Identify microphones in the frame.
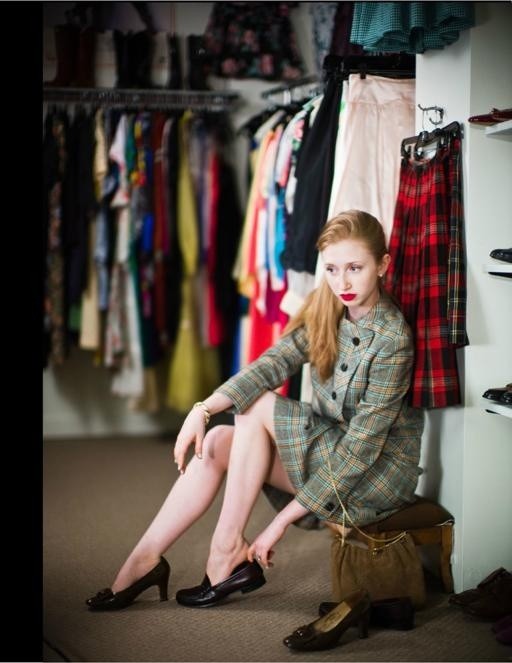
[326,498,453,595]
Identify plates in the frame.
[482,119,512,420]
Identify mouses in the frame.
[400,107,460,157]
[267,52,416,110]
[47,92,231,117]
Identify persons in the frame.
[86,209,425,612]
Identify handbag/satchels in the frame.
[195,401,210,426]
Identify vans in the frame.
[84,555,171,612]
[282,595,372,652]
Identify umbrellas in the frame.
[490,248,511,263]
[466,107,511,126]
[482,383,512,408]
[447,567,512,652]
[175,559,268,610]
[319,595,416,631]
